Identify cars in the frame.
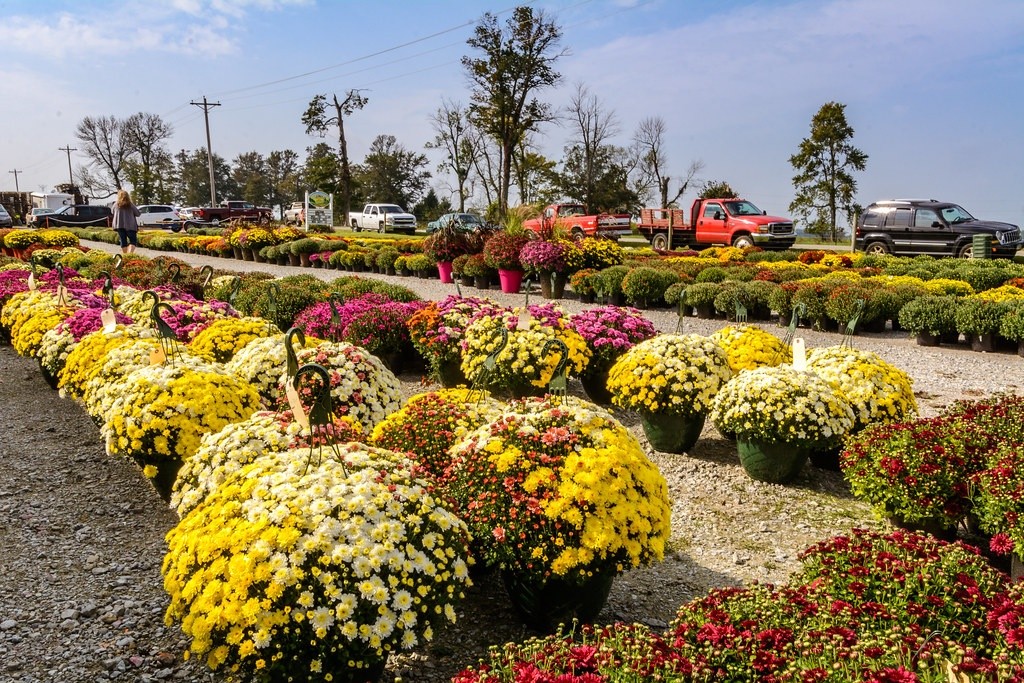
[25,208,54,228]
[425,213,505,236]
[0,203,14,229]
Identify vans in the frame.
[283,202,315,226]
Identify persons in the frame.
[111,191,141,255]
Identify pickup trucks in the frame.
[348,203,418,235]
[521,203,633,243]
[199,200,274,225]
[636,198,797,251]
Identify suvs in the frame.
[850,198,1024,263]
[29,205,114,229]
[135,204,199,232]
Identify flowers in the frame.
[581,361,614,404]
[0,218,1024,683]
[438,359,475,390]
[309,254,319,261]
[508,382,560,401]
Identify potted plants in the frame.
[571,265,1024,351]
[451,253,498,289]
[329,249,429,277]
[437,262,454,283]
[173,235,228,257]
[259,236,320,268]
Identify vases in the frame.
[242,248,253,261]
[503,564,618,636]
[498,269,523,293]
[252,244,266,262]
[133,452,185,505]
[539,267,568,298]
[736,433,804,483]
[641,408,706,454]
[234,247,243,259]
